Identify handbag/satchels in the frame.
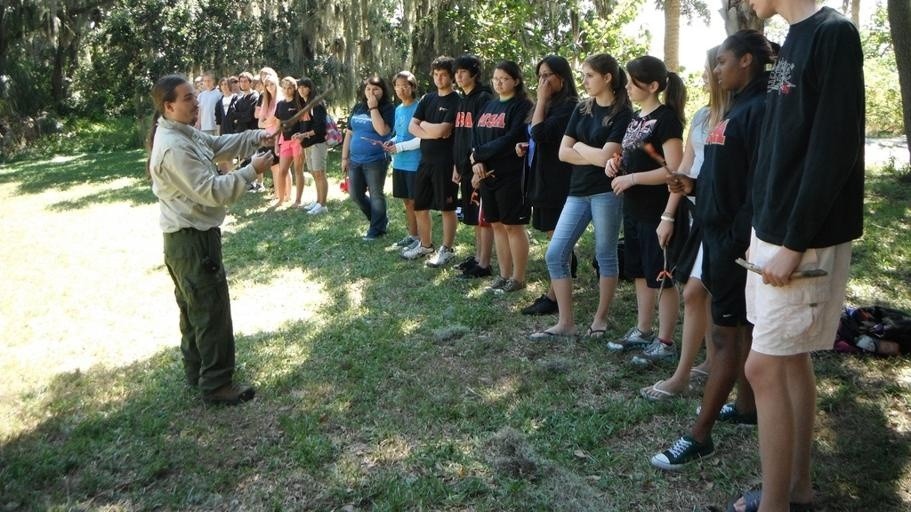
[324,113,342,147]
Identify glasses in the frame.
[491,78,512,82]
[393,85,411,90]
[538,73,554,78]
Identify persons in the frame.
[340,75,389,240]
[637,39,727,402]
[519,54,580,316]
[379,71,422,258]
[724,0,865,512]
[526,54,628,341]
[452,54,491,281]
[146,75,280,407]
[405,54,459,267]
[469,62,532,294]
[149,66,332,219]
[610,56,688,370]
[650,24,775,474]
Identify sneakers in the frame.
[246,182,327,214]
[202,385,253,405]
[362,235,558,315]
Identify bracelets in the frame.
[365,105,379,113]
[657,212,676,223]
